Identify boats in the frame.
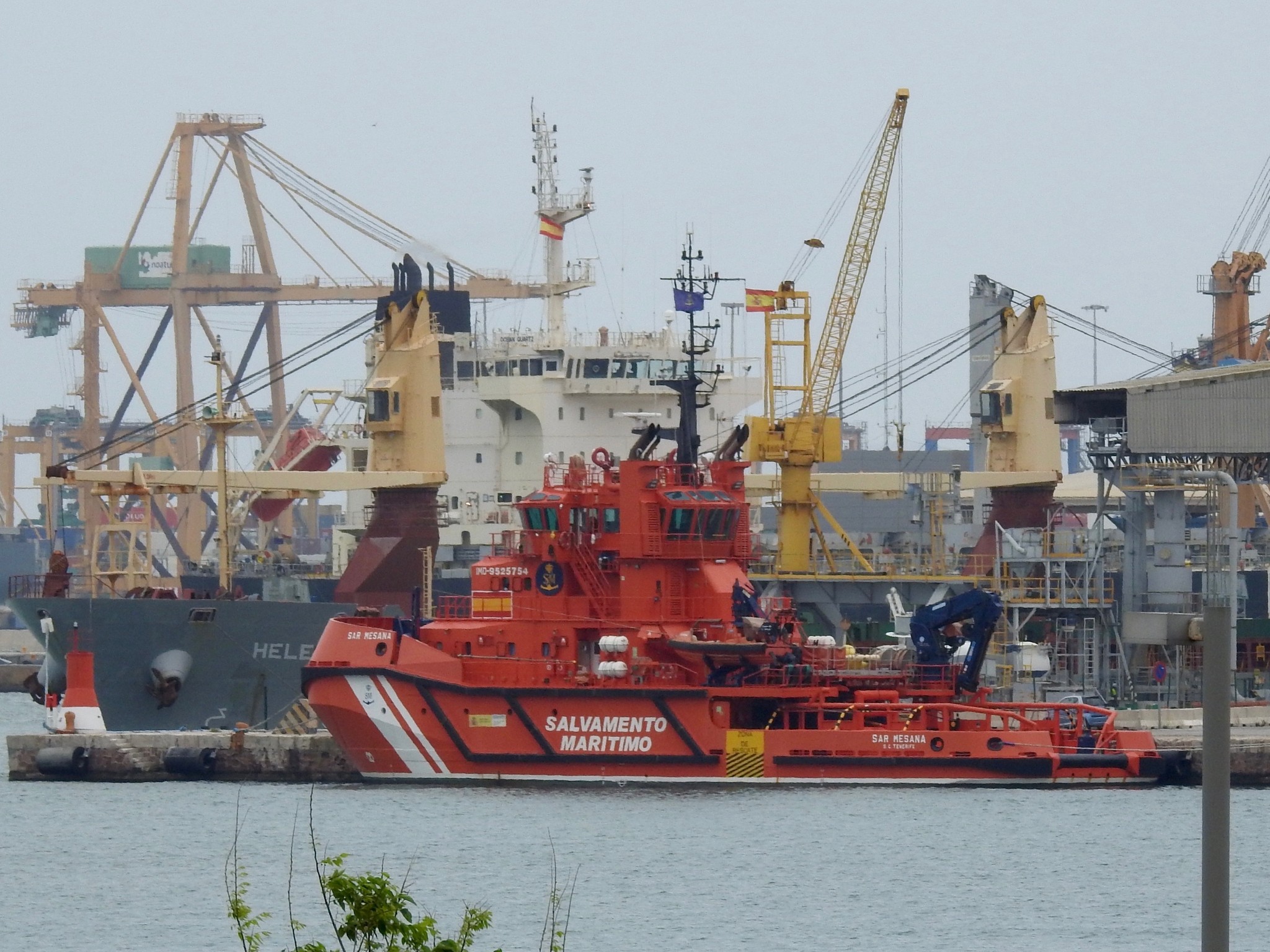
[300,232,1202,791]
[667,596,847,671]
[249,427,340,522]
[0,97,972,732]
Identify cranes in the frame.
[9,109,598,598]
[740,87,912,644]
[909,585,1003,722]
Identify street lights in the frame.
[1080,304,1110,467]
[720,302,745,374]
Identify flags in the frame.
[672,287,704,312]
[745,288,776,312]
[540,216,564,240]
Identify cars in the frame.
[1042,695,1108,730]
